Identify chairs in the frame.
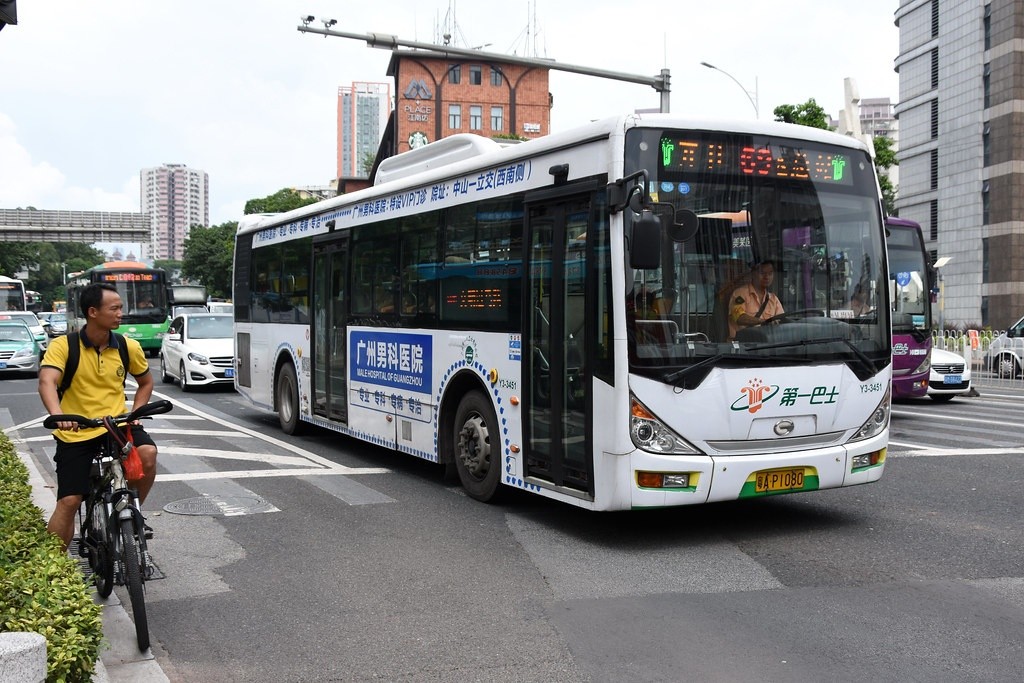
[265,259,416,313]
[715,260,747,343]
[652,288,678,314]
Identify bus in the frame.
[731,210,941,402]
[0,274,29,312]
[63,259,176,357]
[378,255,587,330]
[25,290,44,312]
[232,112,896,515]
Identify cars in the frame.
[158,312,235,392]
[0,300,68,379]
[925,348,971,404]
[157,283,233,325]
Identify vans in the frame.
[984,315,1024,379]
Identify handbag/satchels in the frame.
[122,424,146,480]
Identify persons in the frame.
[335,272,426,328]
[139,294,154,308]
[726,257,785,343]
[841,284,875,318]
[39,285,158,558]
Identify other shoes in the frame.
[134,523,153,539]
